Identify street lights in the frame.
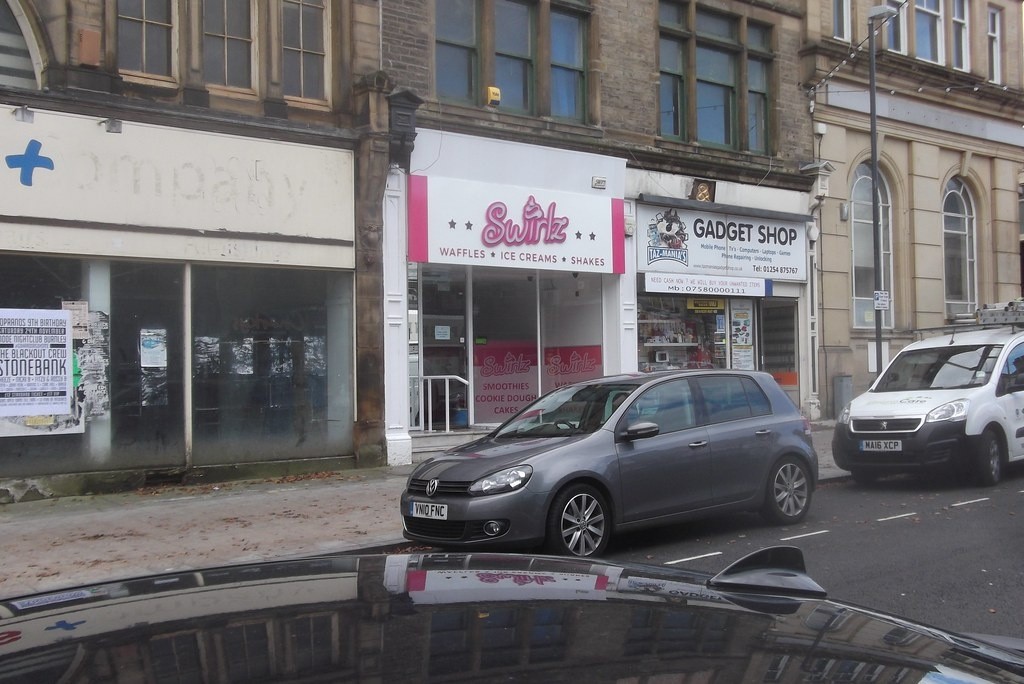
[867,4,899,378]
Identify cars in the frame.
[401,367,818,559]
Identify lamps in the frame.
[97,115,123,134]
[12,104,34,124]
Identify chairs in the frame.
[612,394,636,426]
[653,386,687,431]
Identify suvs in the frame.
[831,301,1024,485]
